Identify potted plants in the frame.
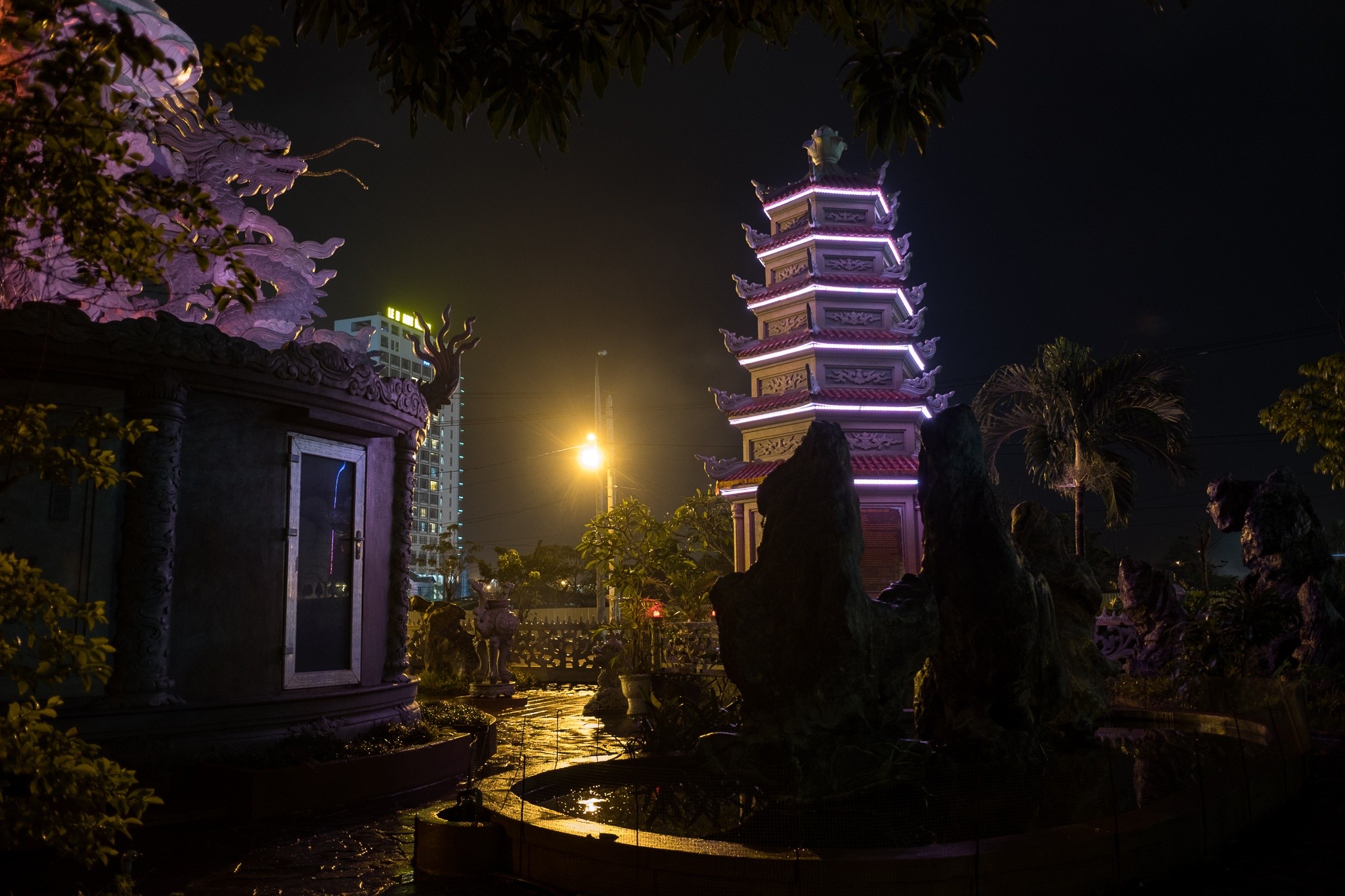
[589,575,672,715]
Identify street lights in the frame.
[580,429,607,618]
